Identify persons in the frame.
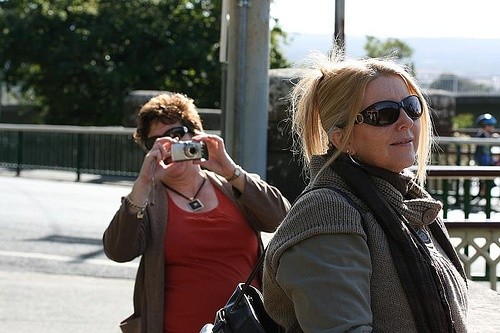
[263,61,468,333]
[102,94,292,333]
[470,115,500,213]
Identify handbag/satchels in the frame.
[201,278,272,333]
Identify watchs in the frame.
[226,166,241,181]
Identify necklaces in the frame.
[161,176,206,211]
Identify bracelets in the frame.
[126,196,148,211]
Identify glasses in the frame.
[144,125,193,150]
[336,94,424,127]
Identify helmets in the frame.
[477,113,497,124]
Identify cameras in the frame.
[171,140,202,162]
